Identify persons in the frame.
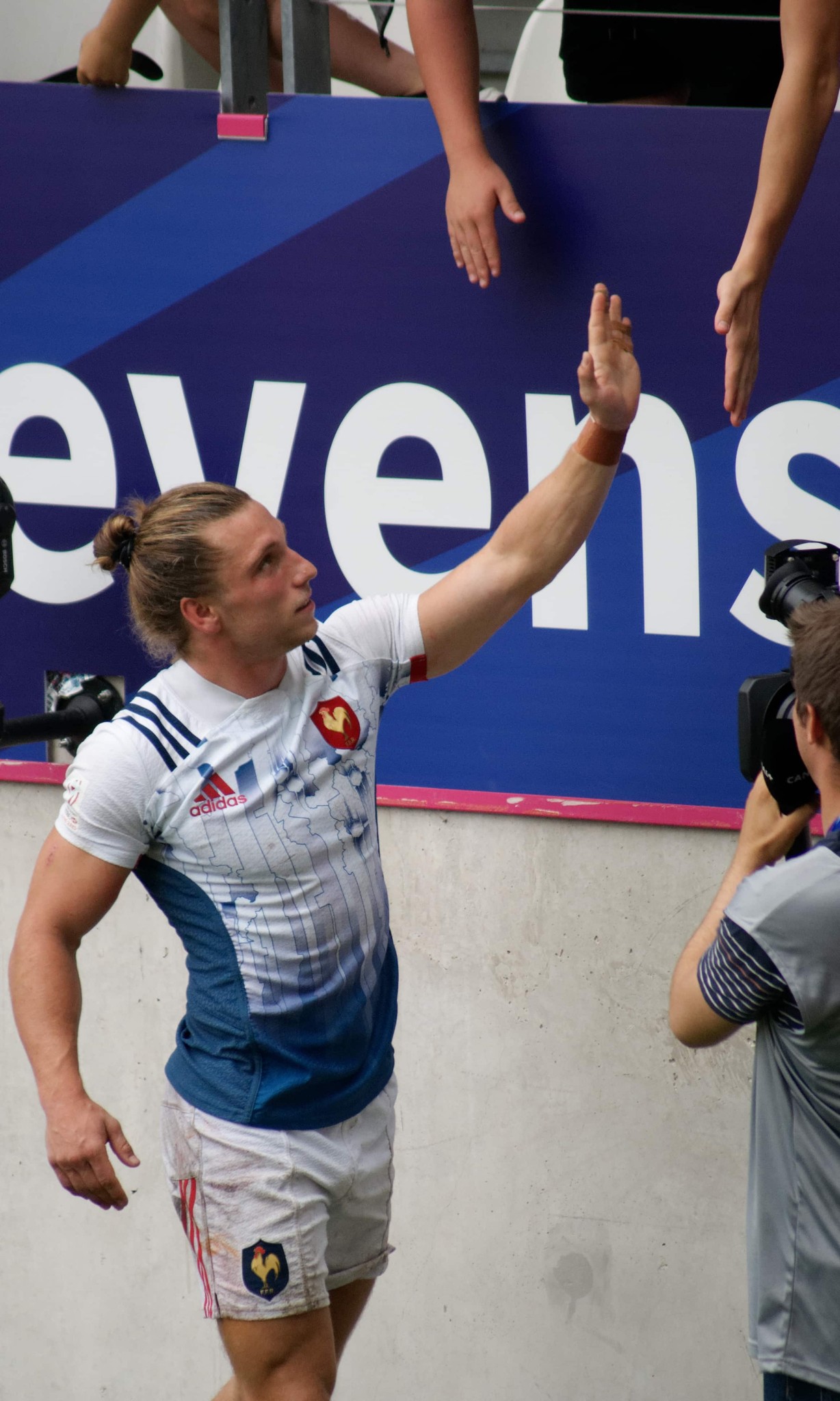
[405,0,840,428]
[9,282,641,1401]
[668,597,840,1401]
[77,0,509,103]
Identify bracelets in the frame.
[574,417,629,466]
